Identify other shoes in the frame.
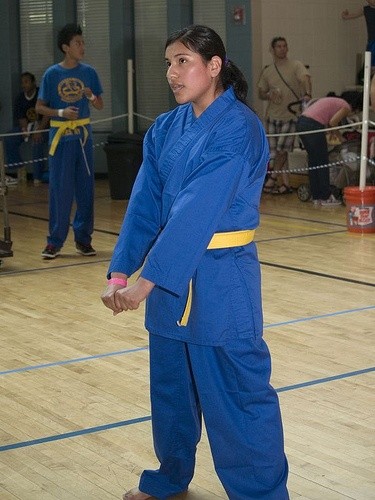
[321,194,342,205]
[34,180,41,184]
[312,199,320,205]
[6,176,19,185]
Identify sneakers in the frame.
[76,241,96,256]
[41,245,60,257]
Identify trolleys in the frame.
[282,97,375,201]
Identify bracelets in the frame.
[304,92,311,97]
[86,93,95,101]
[58,109,63,117]
[265,92,270,98]
[106,277,127,287]
[90,97,97,104]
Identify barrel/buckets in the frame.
[344,188,375,234]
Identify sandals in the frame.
[263,176,277,193]
[272,184,294,195]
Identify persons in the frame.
[296,91,363,205]
[35,24,104,257]
[16,72,50,185]
[100,26,290,500]
[256,37,312,195]
